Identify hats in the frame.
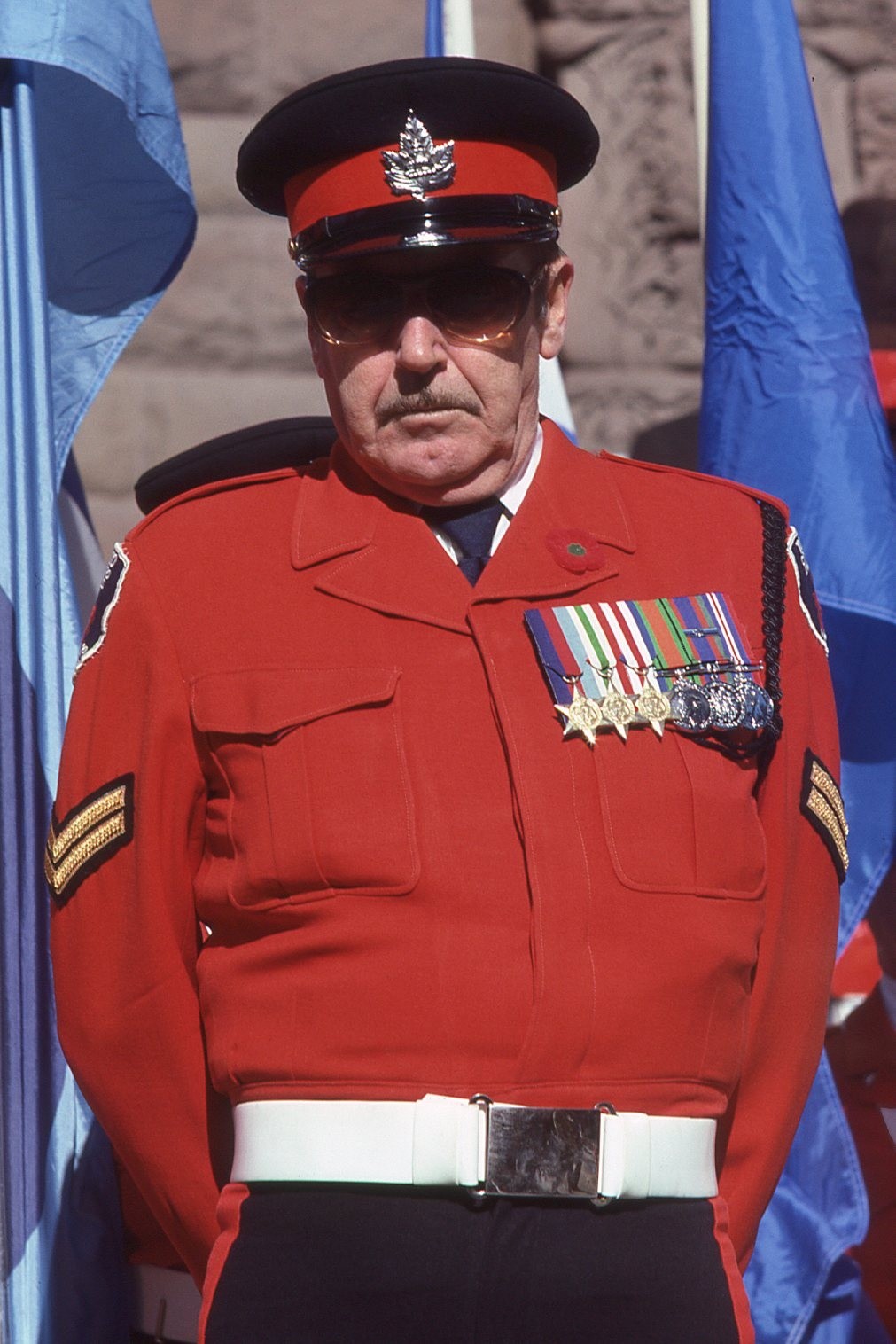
[235,56,600,279]
[134,414,339,514]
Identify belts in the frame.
[227,1094,721,1200]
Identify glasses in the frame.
[304,244,562,346]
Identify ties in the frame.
[420,496,507,585]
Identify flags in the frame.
[699,0,895,1344]
[0,2,202,1343]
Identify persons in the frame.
[0,0,896,1344]
